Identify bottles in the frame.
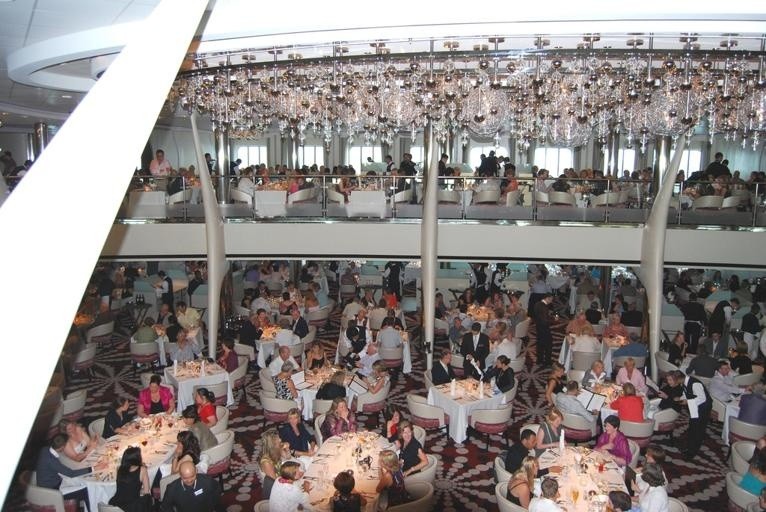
[478,381,485,400]
[224,314,243,329]
[134,293,144,304]
[450,378,456,396]
[558,428,565,451]
[172,359,178,376]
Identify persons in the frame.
[30,258,765,512]
[1,149,33,192]
[531,165,654,203]
[439,150,518,203]
[205,152,417,202]
[126,150,200,205]
[677,152,766,210]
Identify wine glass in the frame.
[570,487,580,510]
